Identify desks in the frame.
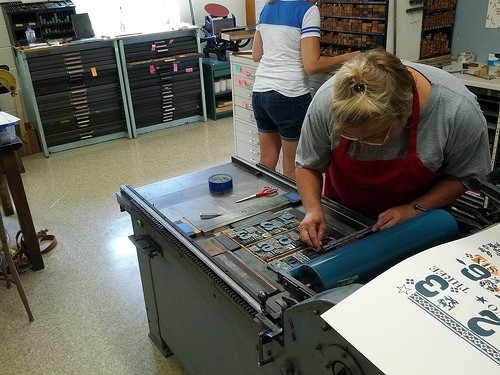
[0,137,45,271]
[432,57,500,209]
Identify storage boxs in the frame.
[15,121,41,156]
[0,111,21,146]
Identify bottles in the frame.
[25,26,36,49]
[456,52,466,65]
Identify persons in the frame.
[294,49,492,253]
[252,0,360,180]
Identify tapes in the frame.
[209,174,232,191]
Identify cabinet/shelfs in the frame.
[201,55,233,120]
[15,38,134,159]
[1,0,77,46]
[119,27,207,135]
[229,49,343,182]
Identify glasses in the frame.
[339,116,397,147]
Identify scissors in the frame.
[236,186,278,203]
